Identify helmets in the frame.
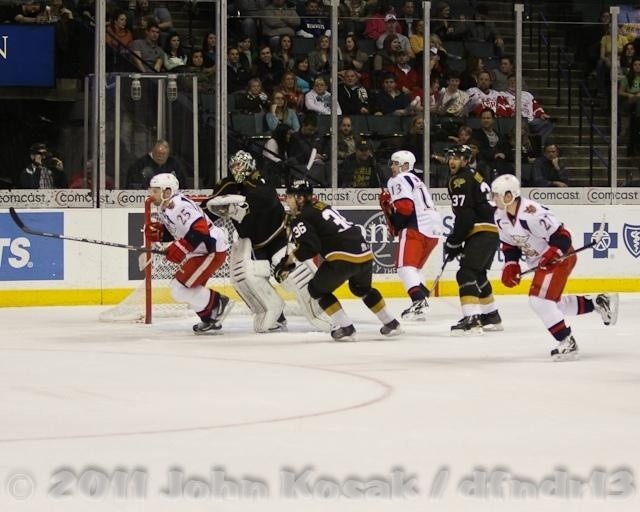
[491,175,521,204]
[150,173,179,201]
[391,151,416,170]
[229,150,257,184]
[285,179,312,194]
[447,144,474,164]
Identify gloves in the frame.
[144,222,165,242]
[538,248,565,272]
[165,238,193,264]
[501,261,520,287]
[443,240,462,261]
[274,255,295,283]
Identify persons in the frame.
[379,150,443,317]
[444,144,501,331]
[150,173,230,332]
[274,180,400,340]
[492,174,611,356]
[199,150,338,333]
[0,0,575,190]
[585,11,640,157]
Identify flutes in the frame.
[10,207,168,254]
[284,148,316,259]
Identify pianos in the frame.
[522,214,607,276]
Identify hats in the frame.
[356,139,372,150]
[29,142,46,150]
[384,12,396,23]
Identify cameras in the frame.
[39,152,57,170]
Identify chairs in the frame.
[73,0,638,188]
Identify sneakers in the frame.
[331,324,355,339]
[451,310,501,330]
[551,335,578,355]
[193,320,223,331]
[401,297,428,317]
[209,295,229,323]
[381,320,399,335]
[595,293,611,325]
[268,316,286,330]
